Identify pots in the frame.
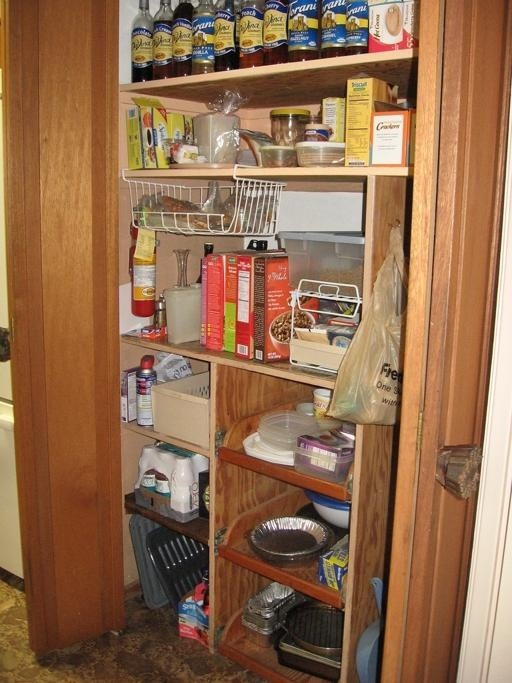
[277,599,345,655]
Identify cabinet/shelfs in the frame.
[118,46,419,683]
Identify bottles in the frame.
[154,301,167,328]
[130,0,369,83]
[196,243,215,285]
[133,441,210,524]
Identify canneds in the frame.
[269,109,310,146]
[304,123,331,141]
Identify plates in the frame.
[385,4,402,36]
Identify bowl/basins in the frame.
[258,142,345,167]
[143,127,154,147]
[303,488,351,530]
[269,311,316,354]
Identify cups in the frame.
[313,388,343,432]
